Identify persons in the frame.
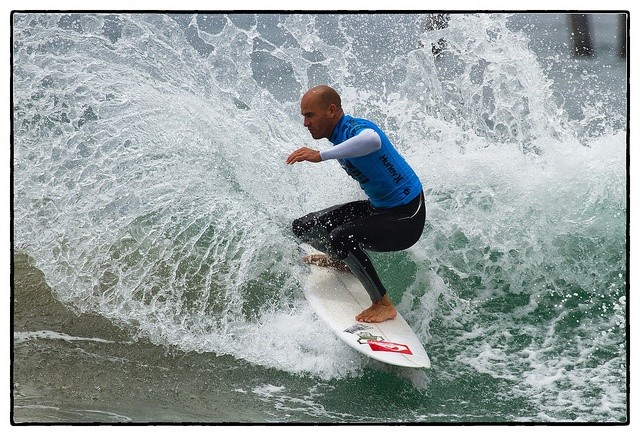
[285,85,425,323]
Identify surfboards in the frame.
[297,242,432,367]
